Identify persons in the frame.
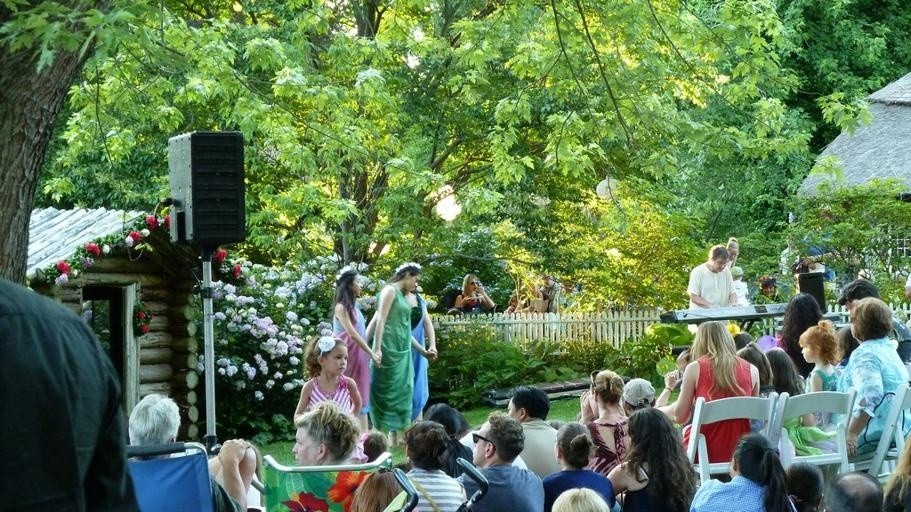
[0,279,127,512]
[128,394,257,512]
[292,235,911,512]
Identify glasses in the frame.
[473,434,496,451]
[591,370,598,387]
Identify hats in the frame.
[623,378,655,407]
[838,279,878,305]
[731,267,744,277]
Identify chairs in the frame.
[855,384,910,487]
[124,443,214,512]
[770,387,857,474]
[686,392,777,485]
[264,451,392,512]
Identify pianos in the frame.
[661,303,789,323]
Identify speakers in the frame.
[798,272,828,314]
[168,131,246,246]
